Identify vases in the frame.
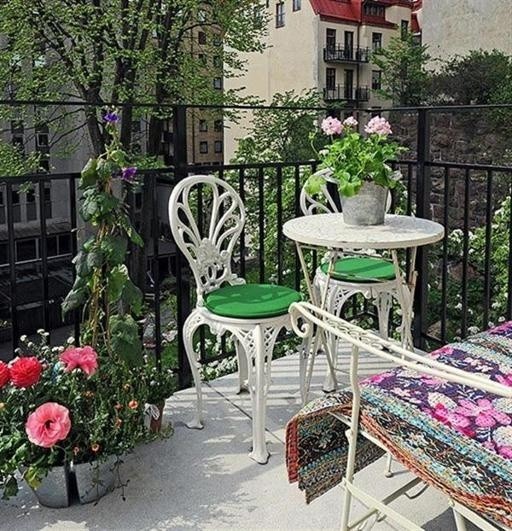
[21,455,121,509]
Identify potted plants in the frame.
[144,367,175,435]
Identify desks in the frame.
[282,214,443,408]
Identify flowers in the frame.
[0,328,142,505]
[316,114,403,180]
